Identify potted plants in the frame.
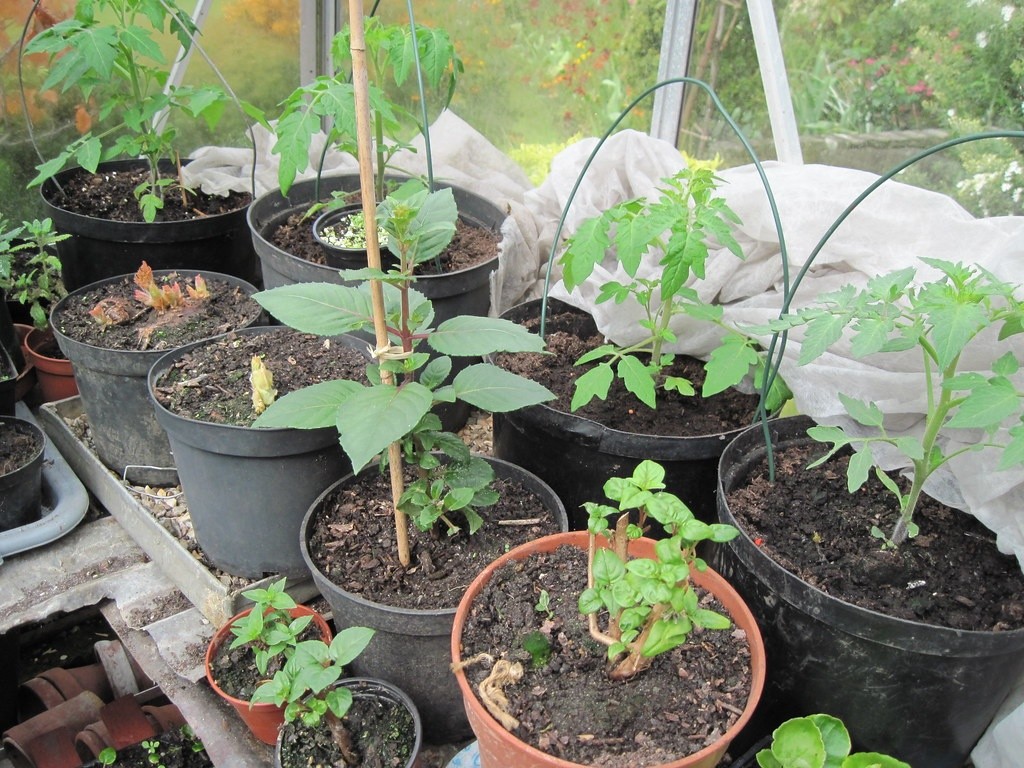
[0,0,1023,768]
